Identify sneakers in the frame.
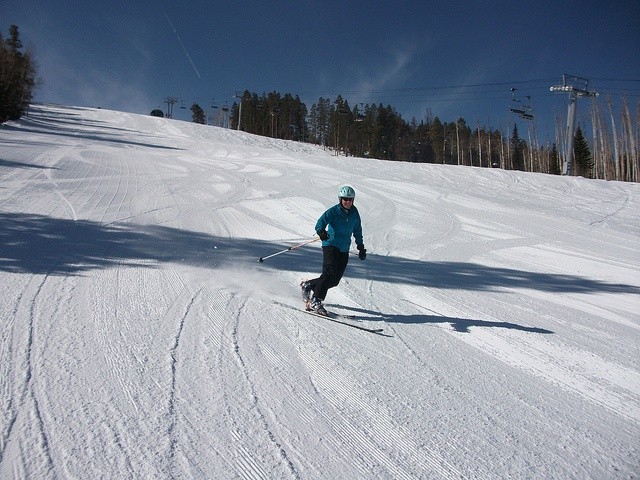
[300,280,311,303]
[312,297,327,315]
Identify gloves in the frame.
[318,230,327,241]
[357,244,366,260]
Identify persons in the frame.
[300,186,367,316]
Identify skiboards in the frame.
[269,293,384,333]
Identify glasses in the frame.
[342,197,354,201]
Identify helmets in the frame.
[338,185,355,202]
